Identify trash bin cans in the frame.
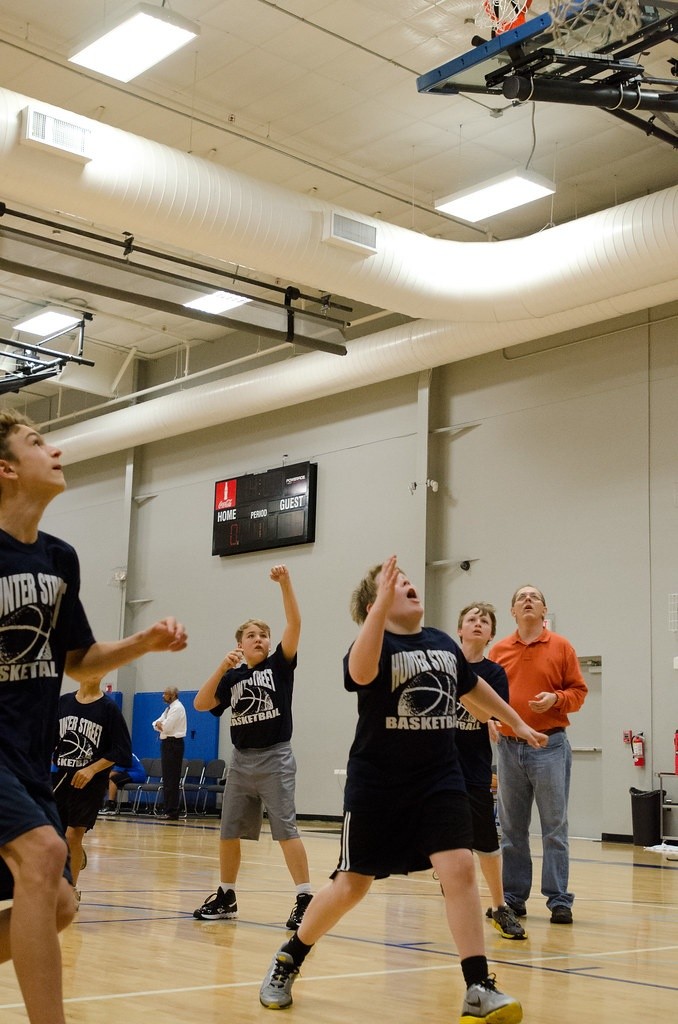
[628,786,667,848]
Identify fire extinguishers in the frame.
[630,732,646,767]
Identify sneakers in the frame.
[459,972,523,1024]
[551,905,572,923]
[193,886,238,920]
[157,814,175,820]
[259,941,303,1010]
[486,902,528,918]
[286,893,313,929]
[97,807,120,816]
[492,905,528,939]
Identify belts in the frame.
[499,727,565,743]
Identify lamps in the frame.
[12,306,84,337]
[68,2,200,83]
[435,167,556,223]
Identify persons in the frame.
[98,753,148,815]
[0,410,187,1024]
[152,687,187,820]
[192,564,314,930]
[259,555,550,1024]
[486,585,588,924]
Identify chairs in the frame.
[117,758,227,818]
